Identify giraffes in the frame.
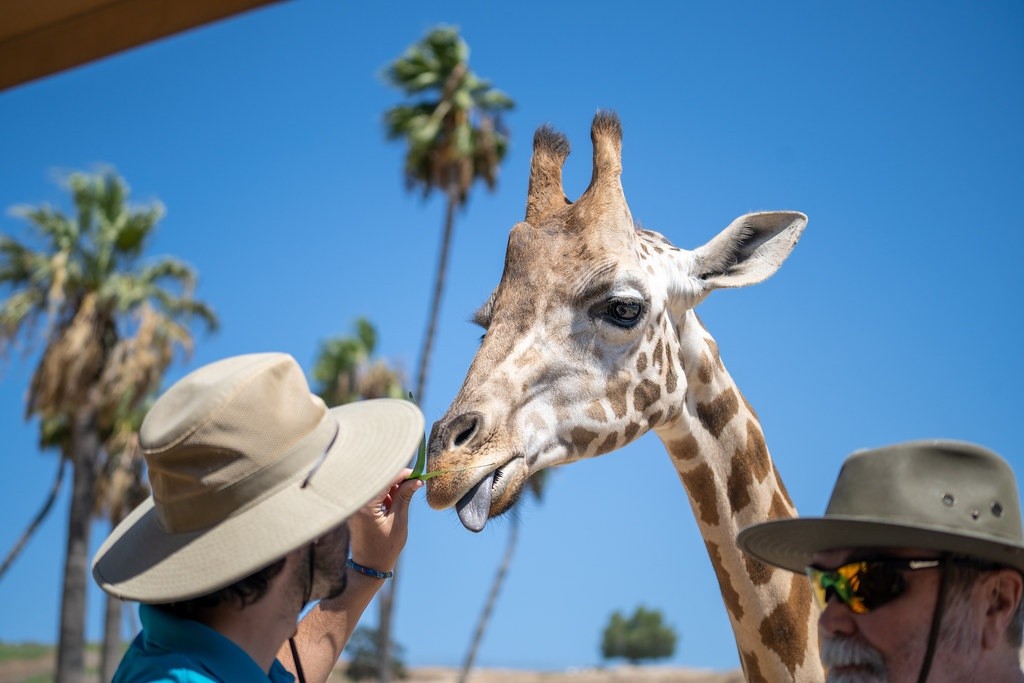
[426,111,824,683]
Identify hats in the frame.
[91,352,427,603]
[736,440,1024,575]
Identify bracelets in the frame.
[348,559,394,579]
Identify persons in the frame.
[735,440,1024,683]
[91,352,424,682]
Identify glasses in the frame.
[804,552,1018,615]
